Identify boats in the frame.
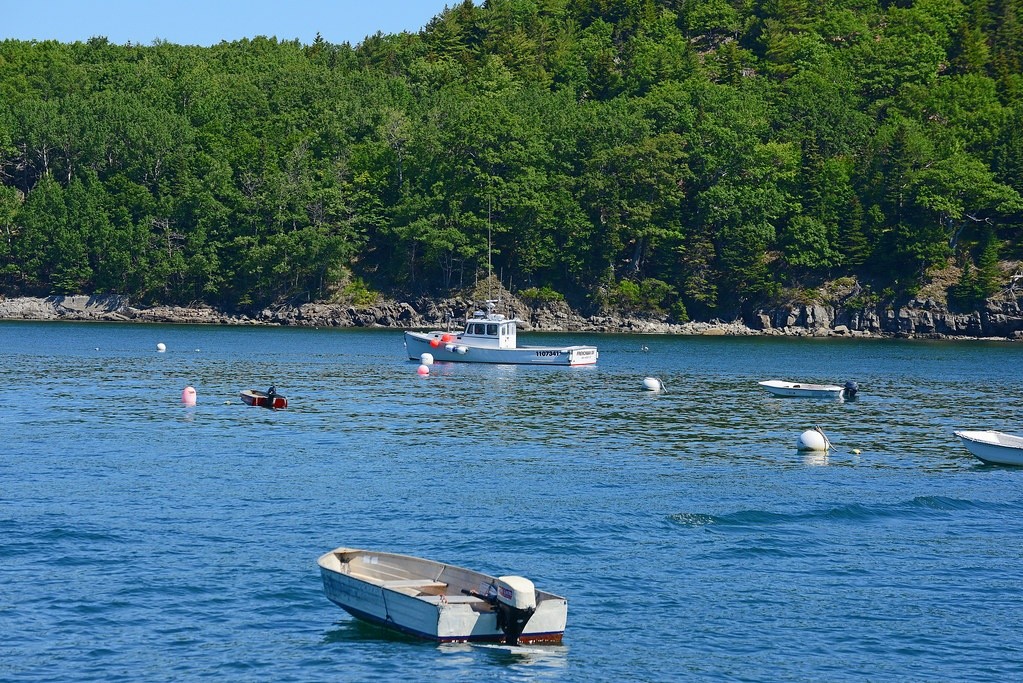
[955,429,1023,465]
[318,548,567,642]
[240,383,287,411]
[758,378,859,398]
[404,193,598,366]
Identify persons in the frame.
[267,386,277,396]
